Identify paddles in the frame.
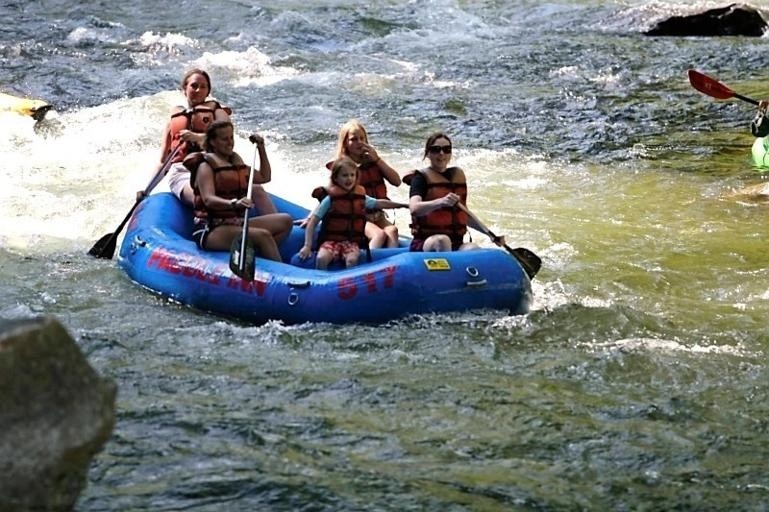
[689,70,760,106]
[229,142,258,282]
[455,201,542,281]
[86,137,185,260]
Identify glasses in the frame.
[429,146,452,154]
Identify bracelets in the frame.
[302,243,312,251]
[230,198,238,210]
[371,157,382,164]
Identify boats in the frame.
[117,182,535,327]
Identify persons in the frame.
[181,120,292,261]
[297,157,409,270]
[291,119,403,250]
[396,131,506,253]
[750,99,769,138]
[134,68,231,217]
